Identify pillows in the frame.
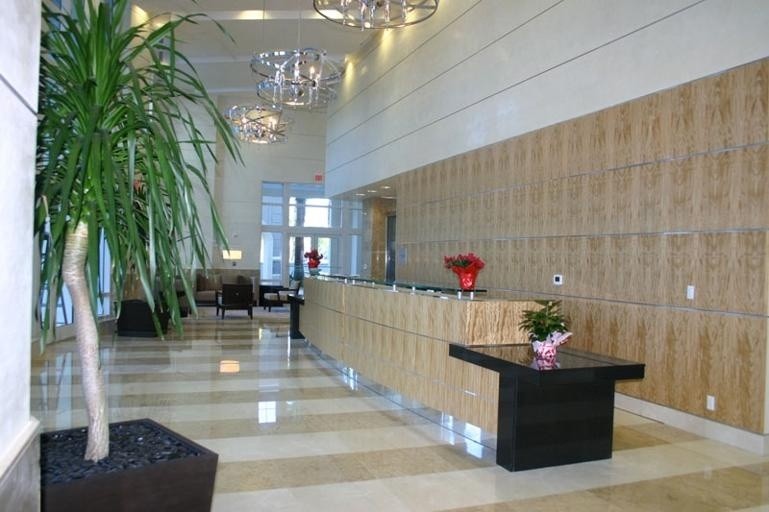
[196,269,256,291]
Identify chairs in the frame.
[264,280,302,312]
[216,284,255,320]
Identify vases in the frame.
[113,300,168,338]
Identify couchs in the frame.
[193,268,260,306]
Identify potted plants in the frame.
[445,251,485,290]
[30,0,245,512]
[304,248,323,268]
[517,299,572,366]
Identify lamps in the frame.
[225,104,297,145]
[251,0,346,113]
[313,0,440,31]
